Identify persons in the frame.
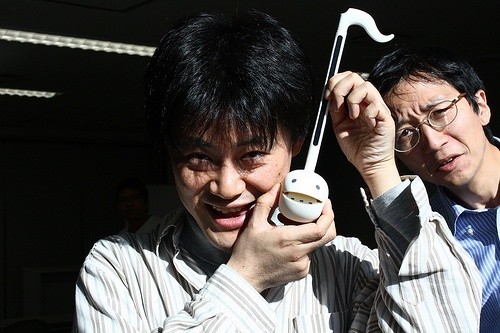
[71,6,483,333]
[361,47,500,333]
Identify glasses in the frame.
[394,91,468,152]
